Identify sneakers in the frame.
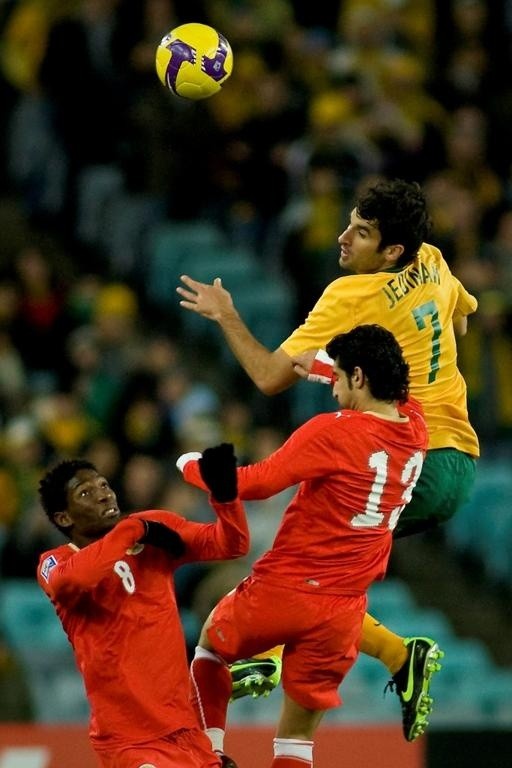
[384,636,444,742]
[227,654,282,703]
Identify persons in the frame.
[181,324,432,766]
[0,0,512,661]
[34,442,252,766]
[174,178,479,741]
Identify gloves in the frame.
[197,443,239,502]
[137,518,186,561]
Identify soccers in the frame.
[156,23,233,98]
